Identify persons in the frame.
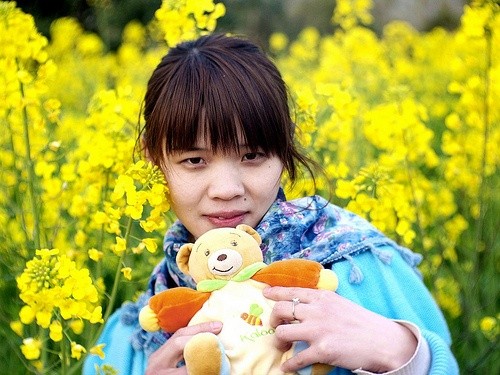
[81,33,461,375]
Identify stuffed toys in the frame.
[138,224,338,375]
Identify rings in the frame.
[291,299,300,320]
